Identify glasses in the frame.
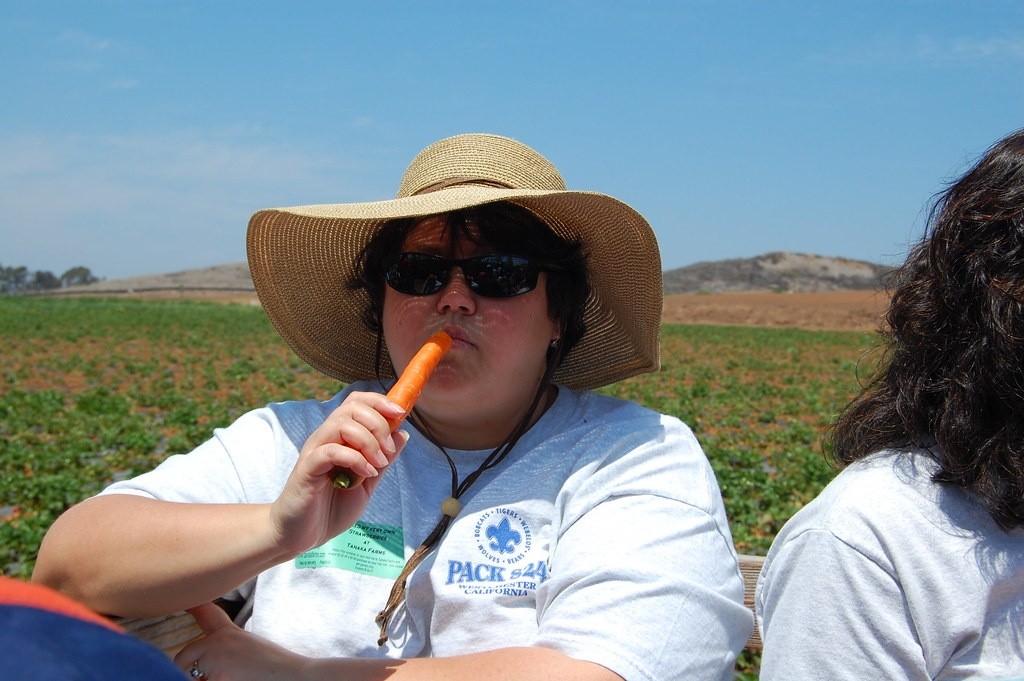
[386,251,539,298]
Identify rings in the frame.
[190,660,207,680]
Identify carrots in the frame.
[328,330,453,490]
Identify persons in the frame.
[30,130,756,681]
[753,123,1024,681]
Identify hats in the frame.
[245,133,663,391]
